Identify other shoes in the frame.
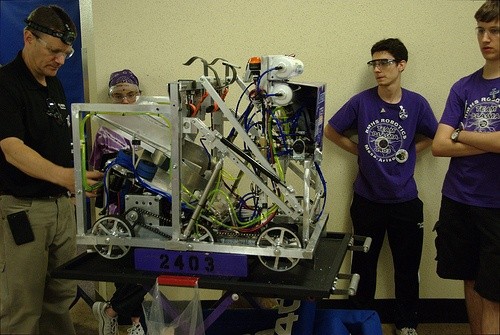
[396,327,417,335]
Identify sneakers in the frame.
[127,321,145,335]
[93,301,119,335]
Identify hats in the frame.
[109,69,139,87]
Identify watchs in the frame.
[450,129,461,142]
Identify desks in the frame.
[50,232,373,335]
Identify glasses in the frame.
[474,26,500,37]
[366,58,396,71]
[30,31,75,59]
[109,90,139,103]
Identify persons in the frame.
[1,4,106,335]
[92,69,159,335]
[432,0,500,335]
[324,38,439,335]
